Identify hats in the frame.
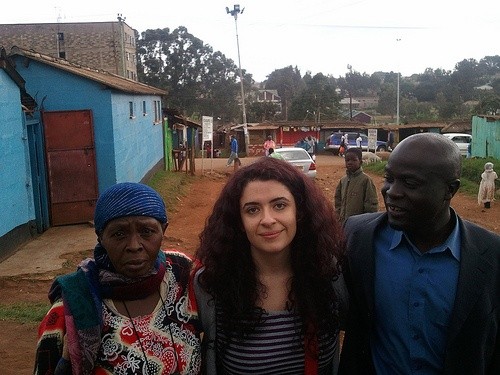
[94,182,168,233]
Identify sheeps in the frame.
[362,152,391,164]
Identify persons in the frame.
[334,147,379,234]
[478,162,498,208]
[193,156,348,375]
[180,130,394,167]
[340,132,500,375]
[32,182,205,375]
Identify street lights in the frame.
[225,3,251,154]
[116,12,127,77]
[392,32,404,125]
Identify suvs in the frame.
[325,132,385,156]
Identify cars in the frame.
[443,132,471,156]
[267,149,316,178]
